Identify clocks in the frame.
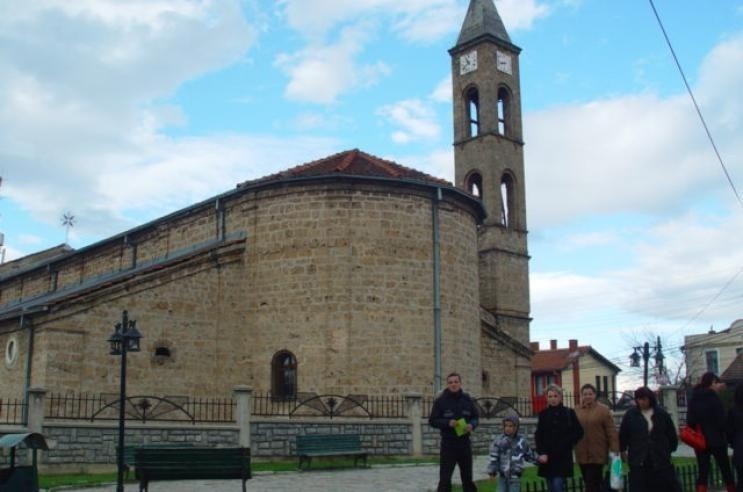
[460,49,478,75]
[497,50,512,76]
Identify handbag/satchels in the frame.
[679,425,707,449]
[609,452,624,490]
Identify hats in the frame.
[503,415,519,425]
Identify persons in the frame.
[429,372,480,492]
[485,373,742,490]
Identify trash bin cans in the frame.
[0,433,50,492]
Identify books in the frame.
[454,418,467,438]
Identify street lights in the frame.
[631,342,663,389]
[108,310,141,491]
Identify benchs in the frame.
[116,444,193,481]
[296,435,370,470]
[135,447,251,492]
